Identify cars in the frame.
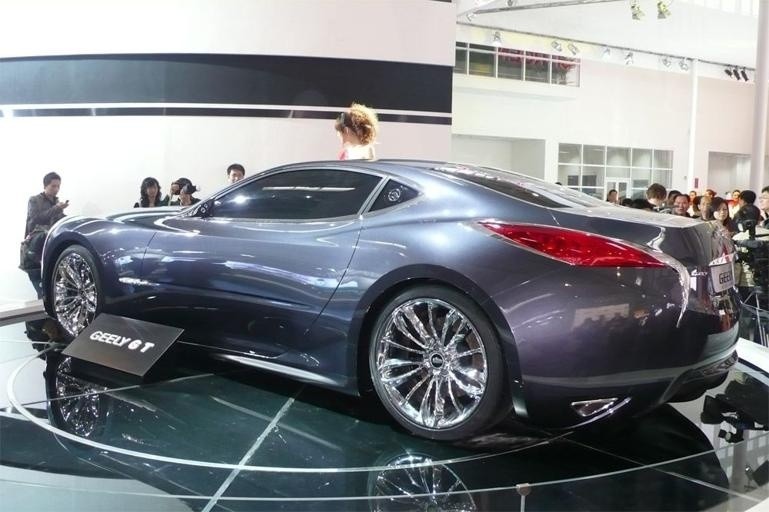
[731,217,768,321]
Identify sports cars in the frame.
[40,158,737,442]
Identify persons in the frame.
[19,172,70,301]
[226,164,245,184]
[334,98,378,160]
[606,183,769,235]
[162,178,200,205]
[135,176,162,207]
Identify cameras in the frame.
[173,183,197,195]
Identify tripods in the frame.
[744,286,769,348]
